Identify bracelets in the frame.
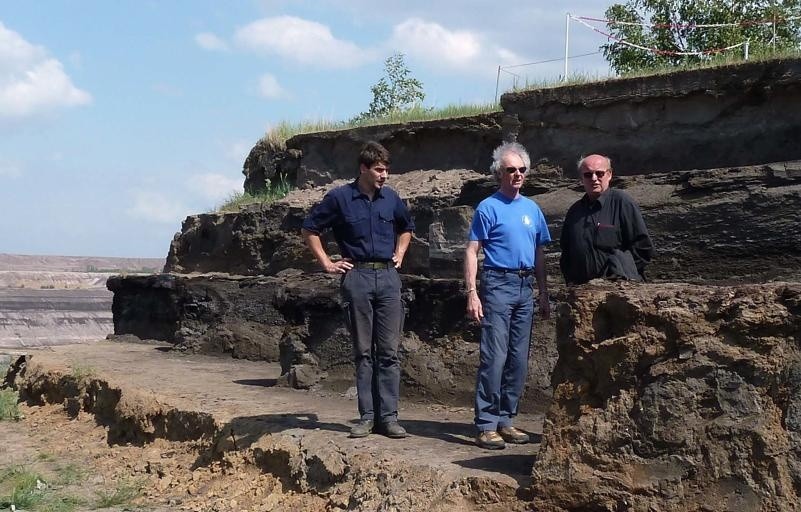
[464,288,478,294]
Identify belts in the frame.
[349,261,398,271]
[483,266,536,277]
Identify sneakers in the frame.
[376,418,406,439]
[350,418,380,438]
[496,426,530,444]
[476,429,506,451]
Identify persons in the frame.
[462,143,553,450]
[301,140,416,437]
[561,153,653,283]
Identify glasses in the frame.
[583,170,610,179]
[506,167,527,174]
